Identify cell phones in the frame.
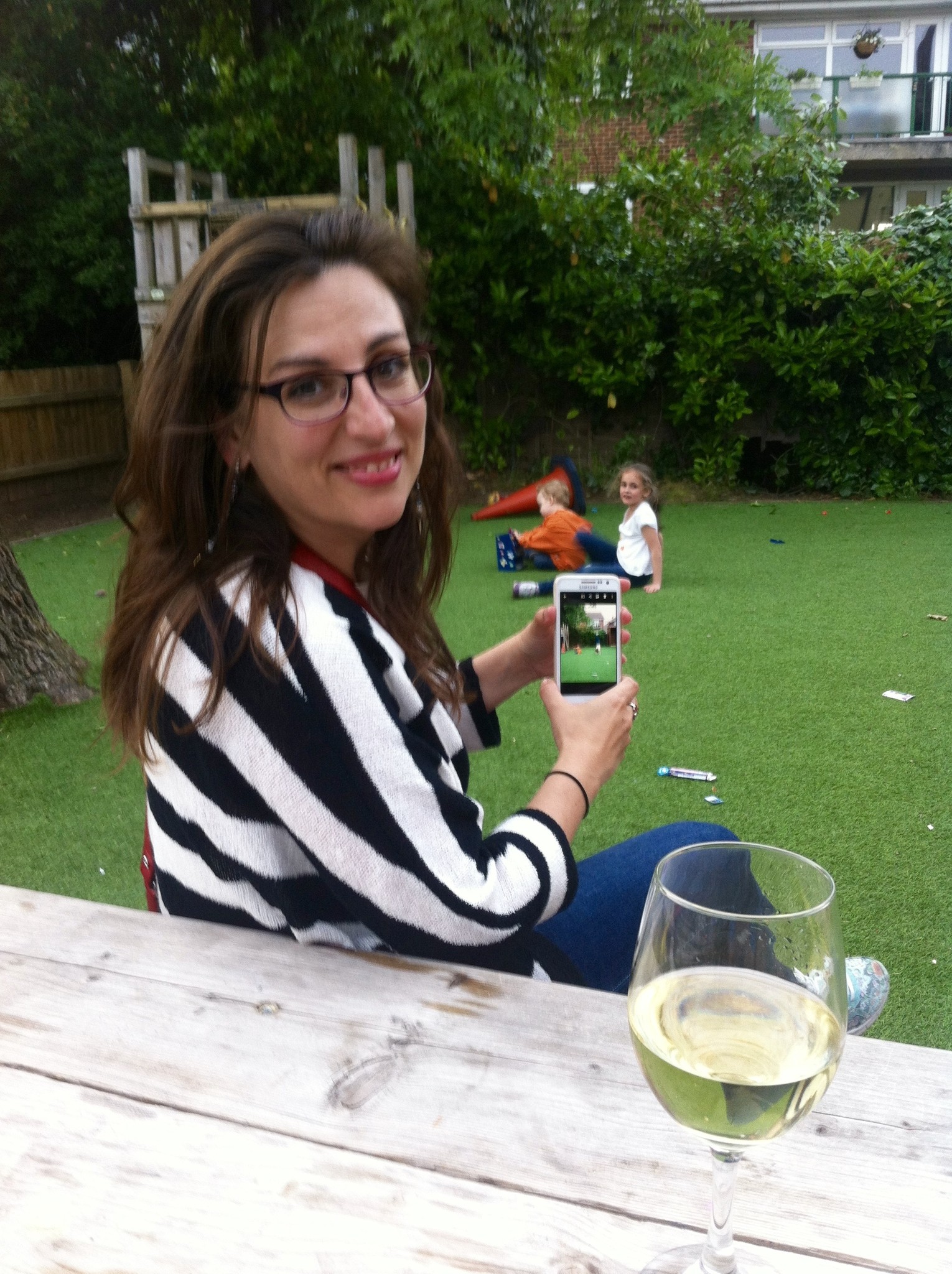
[553,573,622,703]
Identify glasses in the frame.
[241,345,437,427]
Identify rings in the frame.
[630,703,638,718]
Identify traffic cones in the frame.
[467,453,586,521]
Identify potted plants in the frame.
[849,64,886,89]
[787,68,824,91]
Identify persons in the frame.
[572,629,601,653]
[510,481,592,571]
[100,199,892,1037]
[512,462,662,600]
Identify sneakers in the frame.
[513,580,539,598]
[793,957,890,1036]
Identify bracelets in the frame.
[543,771,590,820]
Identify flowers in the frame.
[854,28,886,53]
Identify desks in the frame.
[0,883,952,1273]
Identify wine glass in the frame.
[626,842,846,1274]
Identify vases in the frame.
[854,43,878,55]
